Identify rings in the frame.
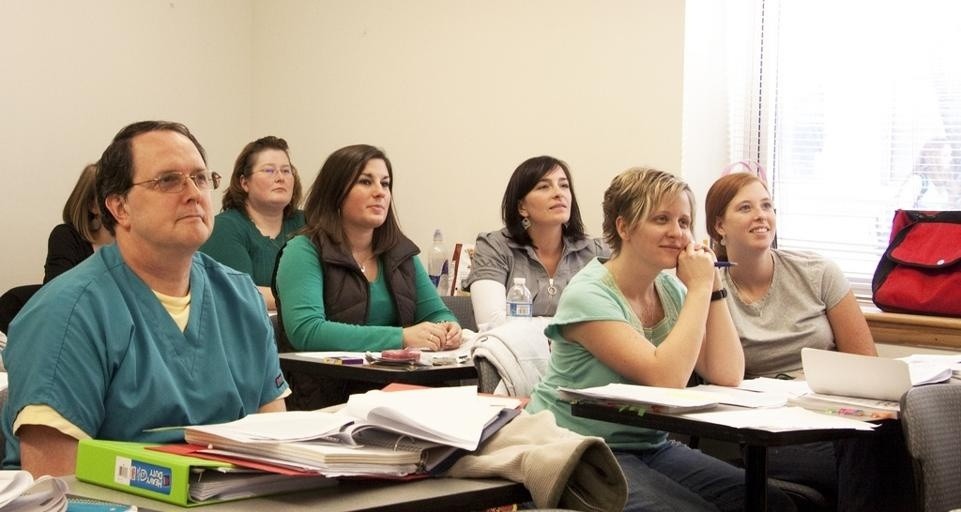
[429,335,434,340]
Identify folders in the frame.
[73,438,338,508]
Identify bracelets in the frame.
[710,289,728,302]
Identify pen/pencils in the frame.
[713,261,739,267]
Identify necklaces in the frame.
[253,220,286,253]
[728,252,775,318]
[350,245,370,275]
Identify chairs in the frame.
[474,357,502,393]
[903,385,961,512]
[441,296,478,333]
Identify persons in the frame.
[706,173,878,512]
[5,121,292,480]
[198,136,308,311]
[463,155,605,332]
[43,162,117,283]
[524,167,747,512]
[276,145,464,352]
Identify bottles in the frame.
[427,228,449,297]
[505,276,533,318]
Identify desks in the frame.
[572,401,902,512]
[266,310,280,330]
[279,353,480,394]
[56,476,533,512]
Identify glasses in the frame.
[132,171,222,191]
[252,167,295,178]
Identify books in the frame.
[75,386,530,508]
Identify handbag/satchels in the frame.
[872,209,961,318]
